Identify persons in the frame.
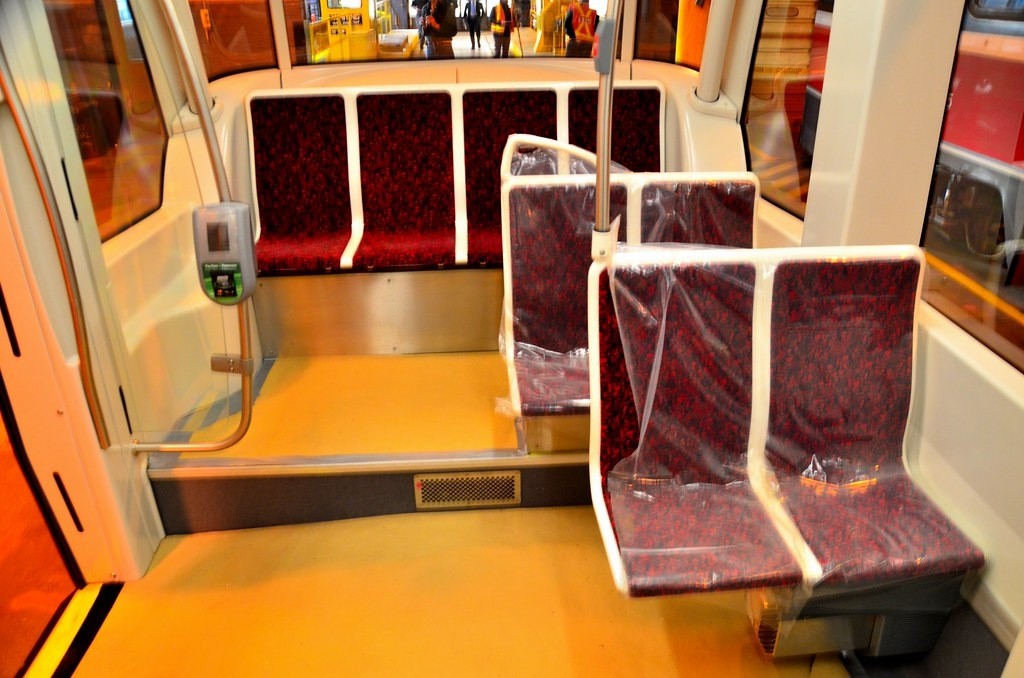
[464,0,484,49]
[565,0,600,57]
[411,0,457,59]
[490,0,515,58]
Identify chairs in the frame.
[236,79,989,661]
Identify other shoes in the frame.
[478,42,481,48]
[472,43,475,50]
